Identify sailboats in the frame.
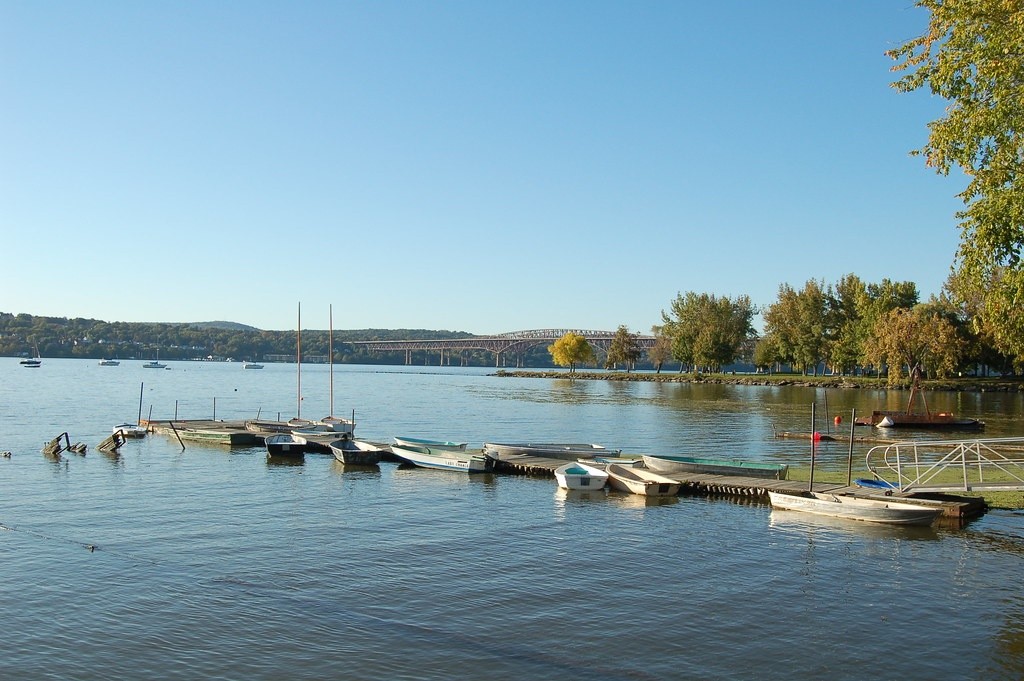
[286,300,314,430]
[143,347,167,368]
[20,335,42,365]
[320,303,357,434]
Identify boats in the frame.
[768,488,946,528]
[553,461,609,491]
[329,436,385,464]
[394,436,468,452]
[264,432,307,456]
[245,364,265,370]
[482,442,622,462]
[113,422,147,438]
[100,359,120,366]
[390,443,488,473]
[640,453,789,480]
[604,462,682,497]
[853,476,902,490]
